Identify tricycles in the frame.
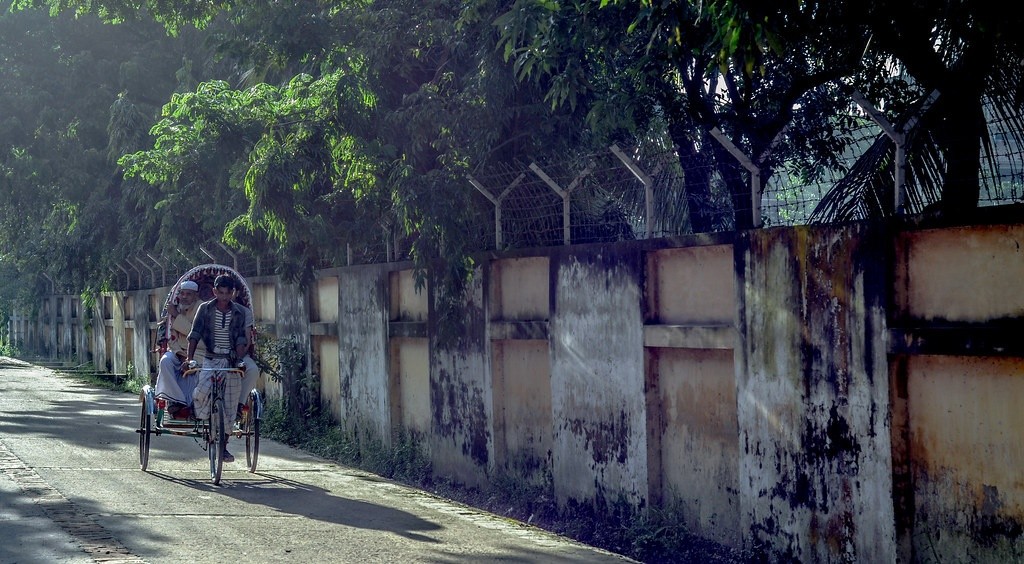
[137,262,263,485]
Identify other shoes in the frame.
[223,449,235,462]
[167,401,183,413]
[190,408,204,421]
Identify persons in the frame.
[153,279,211,414]
[185,275,260,463]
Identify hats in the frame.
[181,281,198,291]
